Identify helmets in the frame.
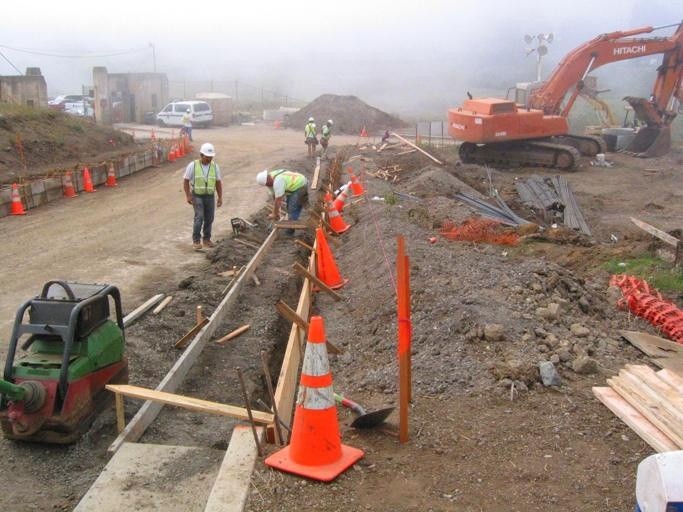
[256,170,267,185]
[308,117,314,122]
[200,143,216,157]
[328,120,333,125]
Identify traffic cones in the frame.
[168,137,185,161]
[105,163,116,187]
[274,120,281,129]
[61,167,77,198]
[310,227,349,290]
[359,125,369,138]
[8,181,27,217]
[323,165,363,232]
[81,167,96,194]
[260,313,366,483]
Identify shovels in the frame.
[334,393,397,429]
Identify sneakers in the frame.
[203,240,215,247]
[193,241,202,250]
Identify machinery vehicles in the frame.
[448,19,682,170]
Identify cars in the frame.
[48,94,83,109]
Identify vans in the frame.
[157,99,212,128]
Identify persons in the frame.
[321,119,334,160]
[182,109,194,142]
[183,142,222,251]
[257,169,308,236]
[305,118,319,156]
